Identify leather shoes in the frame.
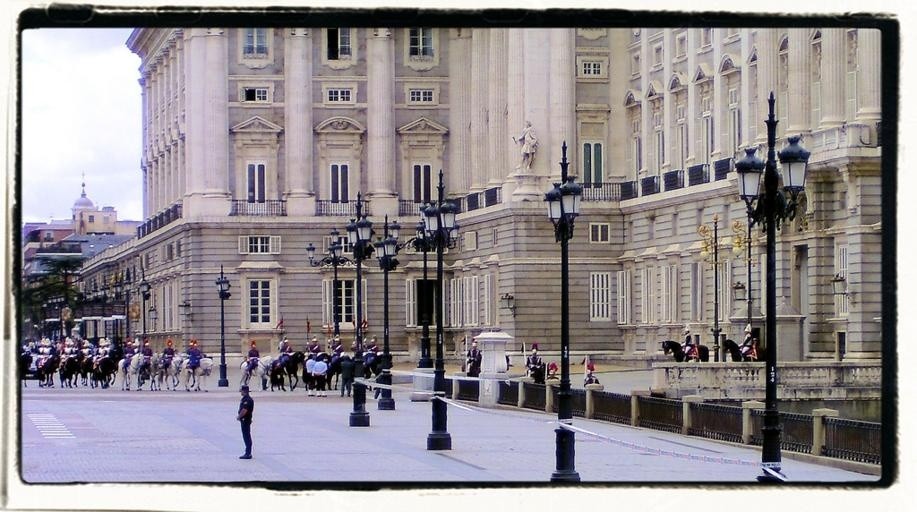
[239,454,251,460]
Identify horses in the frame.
[239,348,392,392]
[722,338,764,362]
[20,351,216,392]
[459,353,482,377]
[529,362,546,384]
[662,340,711,363]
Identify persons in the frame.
[327,336,344,353]
[187,343,194,352]
[234,385,254,459]
[737,326,756,362]
[270,363,286,392]
[123,342,135,366]
[680,327,692,362]
[305,337,321,352]
[280,340,293,353]
[466,342,481,372]
[306,355,315,396]
[583,363,599,388]
[526,349,543,378]
[514,120,539,170]
[190,344,205,374]
[363,339,377,354]
[349,340,359,350]
[546,363,559,380]
[312,357,327,397]
[246,341,258,376]
[24,340,113,372]
[142,343,154,356]
[163,342,174,366]
[340,359,353,396]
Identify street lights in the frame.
[40,276,133,349]
[733,90,811,482]
[137,267,151,347]
[542,139,584,485]
[215,263,232,387]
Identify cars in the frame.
[26,353,45,377]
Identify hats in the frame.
[532,344,538,354]
[684,324,690,331]
[311,336,317,342]
[745,324,751,333]
[239,385,251,393]
[41,336,105,347]
[334,334,340,342]
[251,341,256,348]
[472,343,477,347]
[283,336,288,343]
[127,339,198,347]
[370,335,377,343]
